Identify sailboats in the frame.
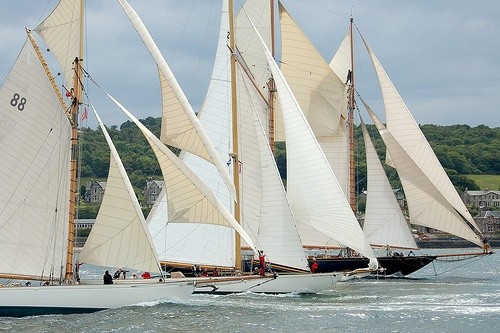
[0,0,497,318]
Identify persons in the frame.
[103,268,150,284]
[312,258,318,274]
[482,237,488,254]
[191,265,220,277]
[385,244,393,256]
[258,249,267,276]
